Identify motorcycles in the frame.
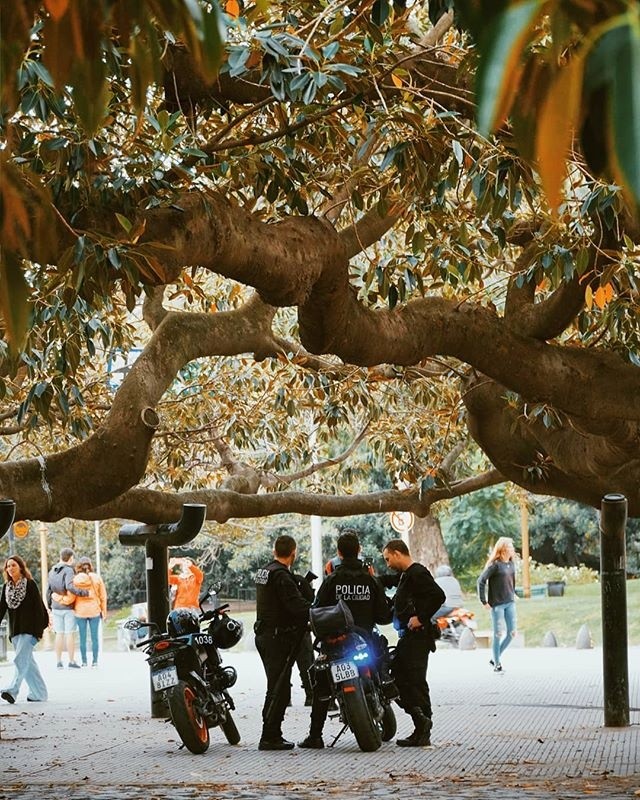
[123,581,241,755]
[432,604,477,650]
[307,598,399,753]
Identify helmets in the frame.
[207,616,245,648]
[166,607,201,636]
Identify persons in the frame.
[382,540,446,747]
[0,556,49,704]
[325,528,375,578]
[168,557,203,610]
[255,536,312,751]
[46,548,108,667]
[296,531,390,750]
[429,564,462,639]
[477,537,517,672]
[284,574,316,707]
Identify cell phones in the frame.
[366,558,373,566]
[174,558,184,564]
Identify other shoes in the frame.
[490,660,495,665]
[27,697,41,702]
[381,673,399,698]
[1,692,15,704]
[259,737,295,750]
[57,661,64,670]
[411,706,432,746]
[297,737,325,749]
[82,662,88,667]
[305,698,314,705]
[494,665,504,676]
[397,733,433,748]
[92,663,98,667]
[69,662,81,669]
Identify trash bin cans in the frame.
[548,581,565,595]
[0,620,7,662]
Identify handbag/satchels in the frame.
[42,601,49,629]
[100,611,104,619]
